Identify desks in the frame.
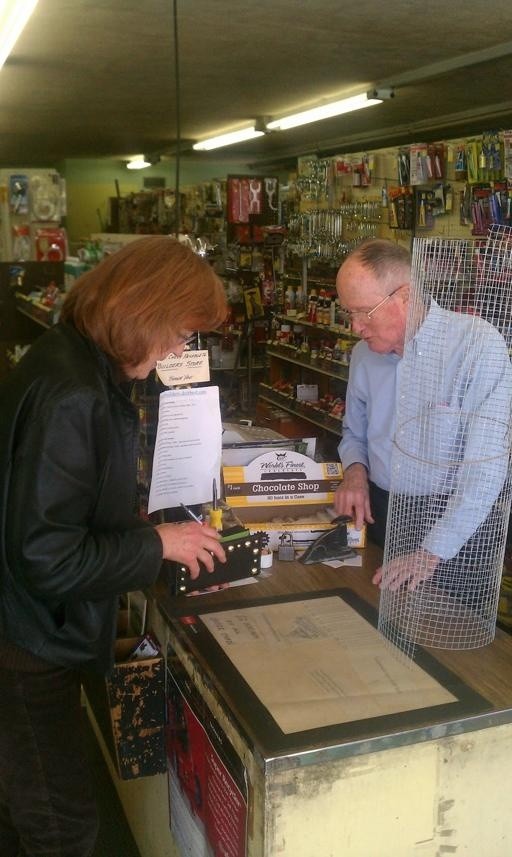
[81,541,511,856]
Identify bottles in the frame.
[282,282,331,325]
[278,322,352,364]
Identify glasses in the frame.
[175,331,195,346]
[338,285,408,322]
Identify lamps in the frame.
[126,154,161,170]
[193,88,396,154]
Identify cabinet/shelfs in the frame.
[259,275,361,437]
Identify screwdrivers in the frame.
[210,479,222,532]
[180,503,208,527]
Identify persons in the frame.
[334,238,511,611]
[0,235,231,856]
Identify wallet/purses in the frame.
[175,532,265,593]
